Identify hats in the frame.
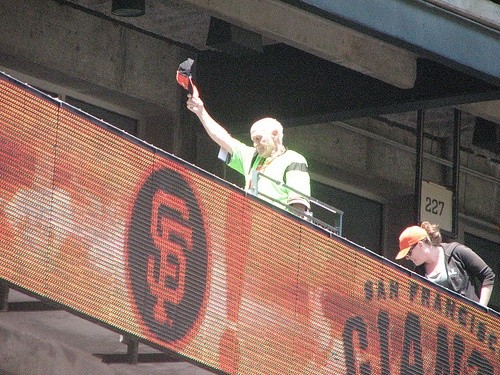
[176,56,201,98]
[396,225,431,262]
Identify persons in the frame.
[185,93,314,223]
[394,221,497,308]
